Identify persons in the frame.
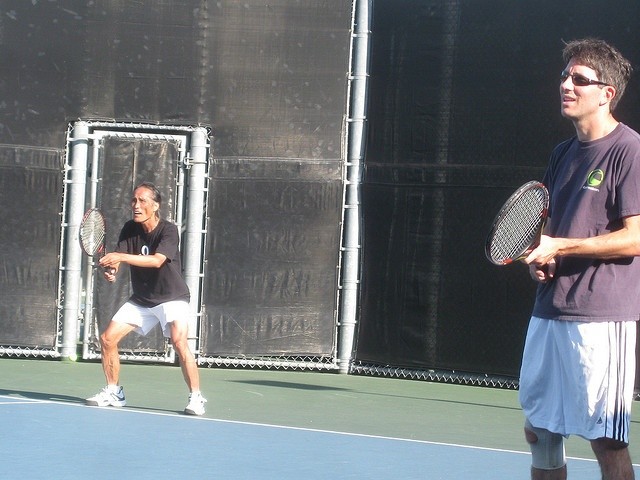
[518,38,640,480]
[86,181,208,416]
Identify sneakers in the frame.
[86,386,126,408]
[183,392,207,415]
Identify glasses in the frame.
[560,70,610,86]
